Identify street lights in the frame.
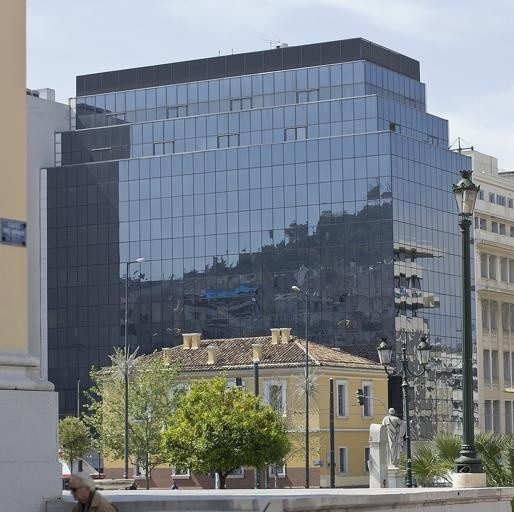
[124,256,148,479]
[292,285,311,490]
[451,169,480,474]
[98,451,100,479]
[377,332,431,486]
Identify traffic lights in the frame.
[356,390,364,405]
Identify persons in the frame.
[128,481,137,489]
[67,470,116,511]
[381,407,402,469]
[170,481,177,489]
